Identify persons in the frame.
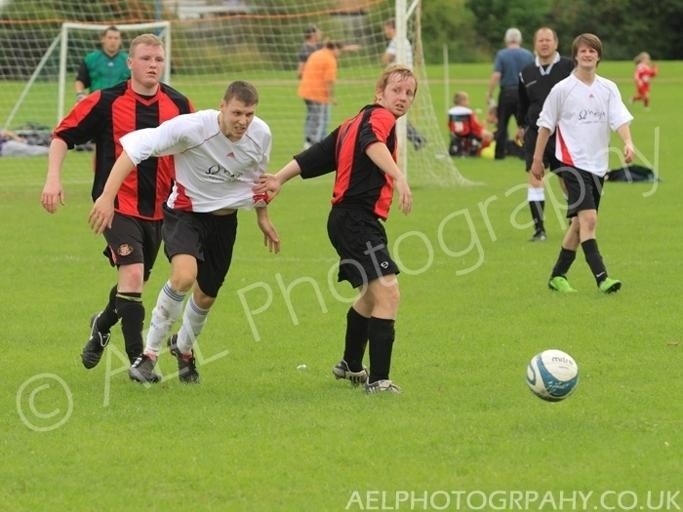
[628,51,657,111]
[298,39,344,149]
[381,17,428,151]
[448,91,493,157]
[40,33,198,383]
[75,26,131,102]
[531,32,635,294]
[252,64,419,395]
[514,26,575,242]
[486,27,535,159]
[296,23,360,82]
[87,81,280,385]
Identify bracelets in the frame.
[75,92,85,99]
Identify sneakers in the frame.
[527,231,548,244]
[361,377,403,396]
[79,312,111,370]
[166,333,201,385]
[332,360,368,386]
[597,276,622,293]
[547,274,578,294]
[126,350,161,383]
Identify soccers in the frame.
[528,350,579,402]
